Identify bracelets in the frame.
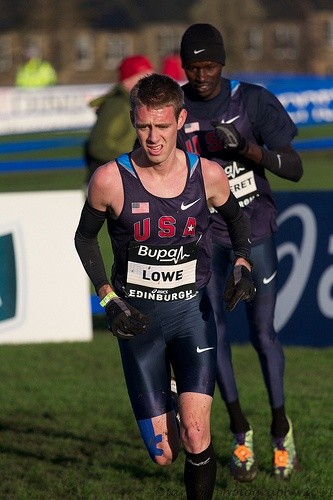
[99,291,118,308]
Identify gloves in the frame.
[100,291,152,340]
[210,121,246,150]
[224,265,256,312]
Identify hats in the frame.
[162,54,185,80]
[118,55,153,81]
[181,24,225,68]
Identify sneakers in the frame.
[170,376,180,423]
[270,414,296,481]
[231,425,257,482]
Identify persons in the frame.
[74,73,256,500]
[134,22,303,481]
[83,55,152,186]
[14,42,58,88]
